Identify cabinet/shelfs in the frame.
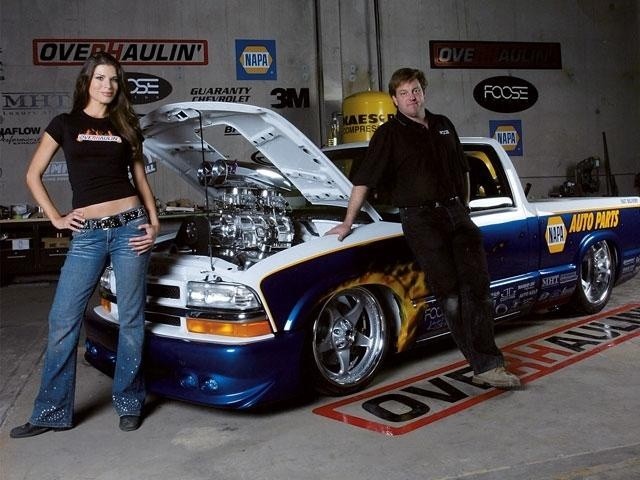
[0,219,72,288]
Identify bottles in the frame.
[328,113,339,146]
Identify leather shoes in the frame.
[119,414,140,431]
[10,422,73,438]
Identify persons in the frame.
[320,67,523,390]
[5,49,162,439]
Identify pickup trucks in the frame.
[81,103,639,414]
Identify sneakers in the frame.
[472,365,521,387]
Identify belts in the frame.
[71,206,146,229]
[400,201,436,207]
